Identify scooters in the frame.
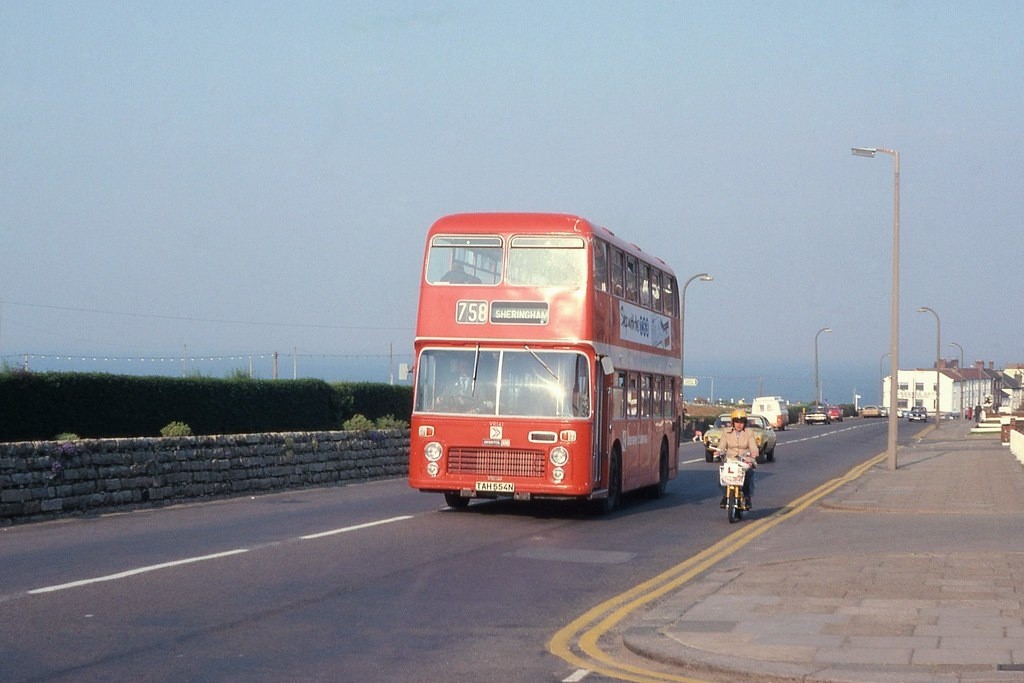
[713,455,752,522]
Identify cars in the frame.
[804,405,843,424]
[861,404,908,418]
[704,411,776,464]
[907,406,927,422]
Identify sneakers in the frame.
[720,496,727,508]
[744,497,752,510]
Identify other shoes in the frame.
[699,440,703,442]
[692,438,697,443]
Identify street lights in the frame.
[879,353,891,405]
[848,146,900,469]
[680,271,714,441]
[948,342,964,420]
[917,306,940,429]
[814,328,833,405]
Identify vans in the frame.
[751,395,790,431]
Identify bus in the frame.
[398,212,683,515]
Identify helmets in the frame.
[731,410,747,422]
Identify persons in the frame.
[712,410,759,512]
[443,261,471,284]
[682,408,755,441]
[436,361,485,414]
[967,403,985,423]
